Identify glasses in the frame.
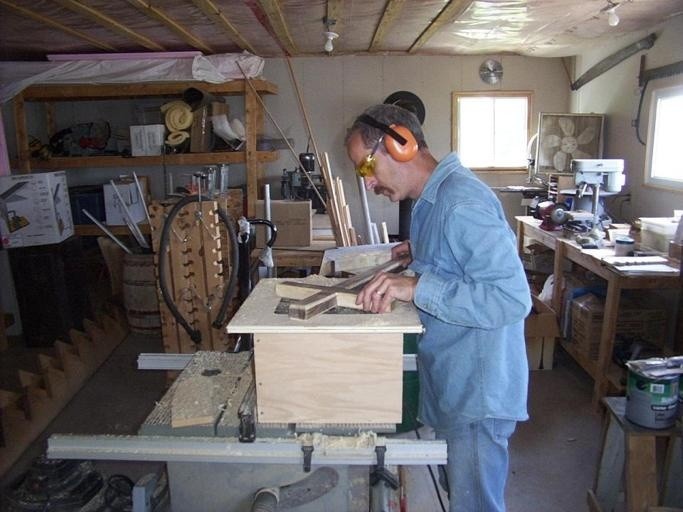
[355,133,386,178]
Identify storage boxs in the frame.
[128,124,169,157]
[0,169,147,250]
[254,199,312,249]
[521,289,668,372]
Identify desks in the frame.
[511,213,682,409]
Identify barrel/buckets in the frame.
[121,250,159,337]
[625,358,679,429]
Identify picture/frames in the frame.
[533,111,605,176]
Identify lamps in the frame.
[601,2,620,27]
[322,31,339,52]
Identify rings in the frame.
[386,292,393,299]
[375,290,384,296]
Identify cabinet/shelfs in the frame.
[13,79,278,289]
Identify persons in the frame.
[339,103,536,511]
